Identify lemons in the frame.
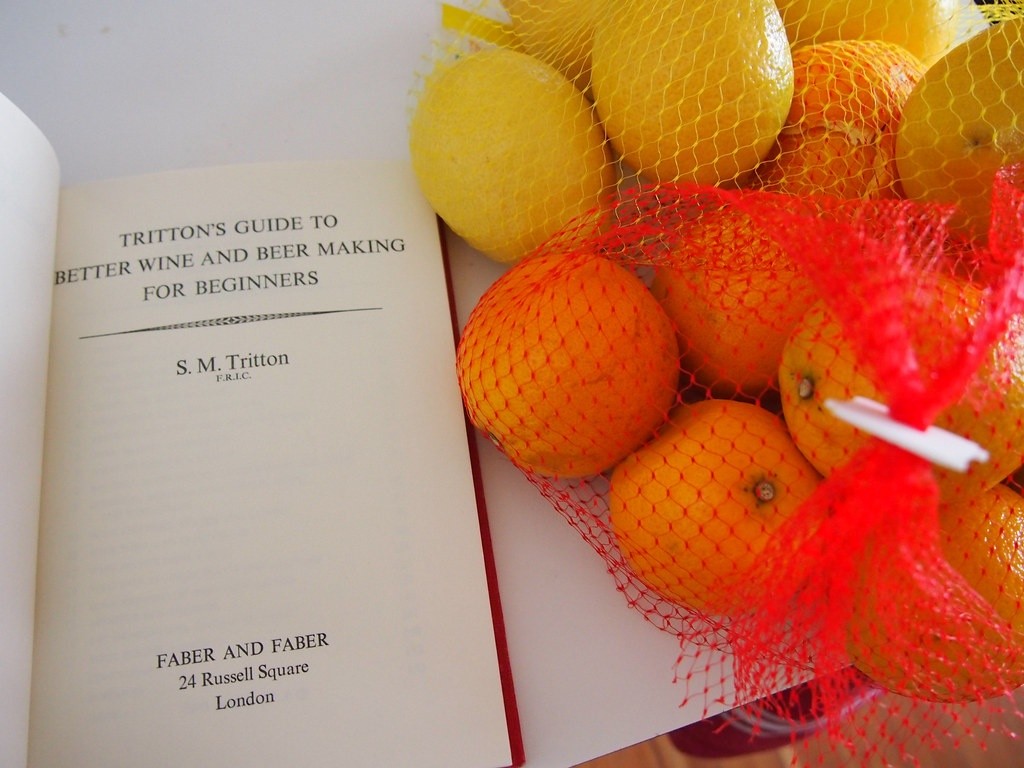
[410,0,1024,263]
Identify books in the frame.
[0,91,524,768]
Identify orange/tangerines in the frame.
[456,41,1024,699]
